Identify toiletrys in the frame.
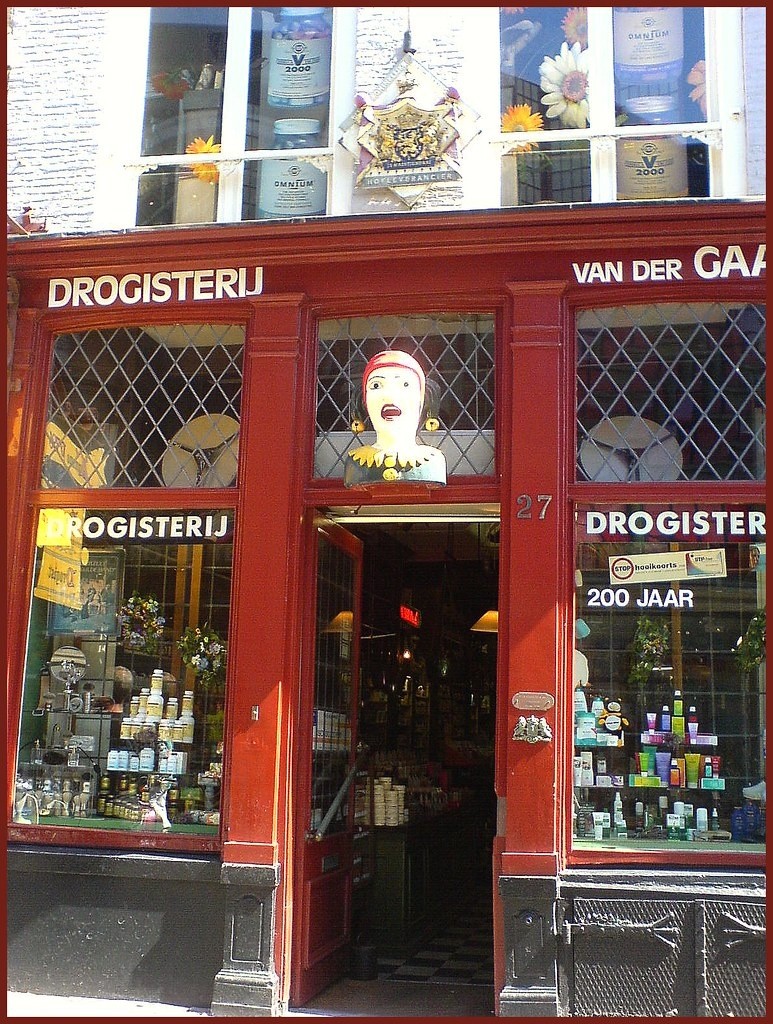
[592,791,628,842]
[628,746,727,790]
[573,686,630,747]
[634,795,720,843]
[572,752,625,789]
[640,690,718,746]
[730,800,766,844]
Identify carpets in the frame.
[348,890,494,986]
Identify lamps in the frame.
[321,557,353,632]
[469,523,499,632]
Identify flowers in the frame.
[560,6,588,51]
[150,60,198,100]
[501,7,529,14]
[688,60,707,120]
[185,134,221,185]
[501,102,553,204]
[538,42,629,150]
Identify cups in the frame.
[674,802,684,815]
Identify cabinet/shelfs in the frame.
[361,623,492,946]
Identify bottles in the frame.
[635,802,644,830]
[704,758,713,778]
[262,7,332,103]
[688,707,697,723]
[603,807,611,839]
[671,760,680,787]
[661,706,671,731]
[673,690,683,717]
[613,791,623,829]
[106,669,193,775]
[711,807,720,831]
[659,796,667,823]
[255,119,325,221]
[96,771,178,822]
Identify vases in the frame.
[183,88,223,149]
